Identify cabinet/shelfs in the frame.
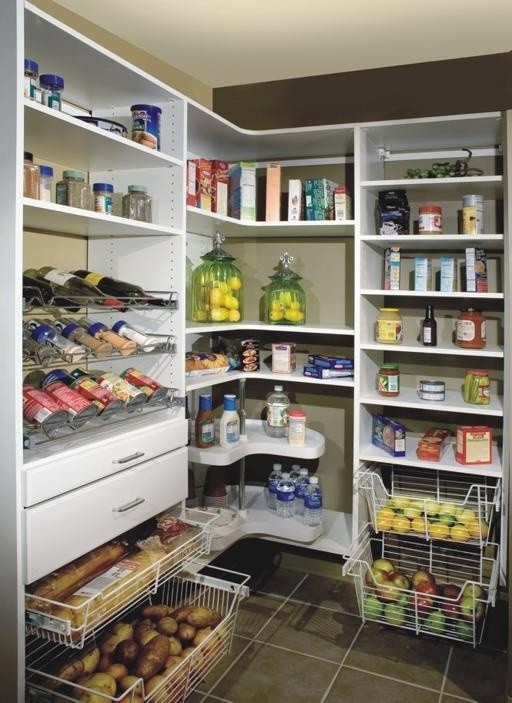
[341,123,509,645]
[20,7,251,702]
[185,147,360,567]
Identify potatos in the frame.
[140,630,160,646]
[163,655,183,669]
[73,673,116,698]
[79,686,111,703]
[130,634,170,680]
[187,606,222,627]
[79,645,99,673]
[101,623,134,655]
[217,625,228,638]
[177,623,193,640]
[193,627,219,652]
[100,653,111,673]
[115,638,137,662]
[181,646,203,675]
[117,694,146,703]
[158,617,177,634]
[104,663,127,680]
[145,675,174,703]
[163,664,189,697]
[41,657,84,691]
[121,676,143,694]
[168,636,183,657]
[142,605,174,619]
[171,606,193,622]
[135,618,157,642]
[131,618,138,626]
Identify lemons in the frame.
[376,497,490,542]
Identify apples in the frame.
[362,558,487,640]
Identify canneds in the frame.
[192,231,244,323]
[264,251,307,325]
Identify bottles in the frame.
[24,59,42,102]
[266,384,290,438]
[462,194,483,235]
[23,384,68,431]
[56,170,91,211]
[455,307,486,349]
[38,74,65,112]
[130,105,163,154]
[303,476,324,527]
[87,322,136,356]
[294,468,309,515]
[289,412,307,444]
[93,184,114,214]
[39,166,54,202]
[42,369,75,390]
[376,308,404,344]
[220,394,241,449]
[418,206,442,235]
[269,464,283,509]
[22,275,81,312]
[39,265,130,314]
[122,184,153,223]
[22,151,41,199]
[112,320,158,353]
[378,365,400,397]
[120,367,169,405]
[75,269,166,308]
[97,372,147,413]
[423,304,438,346]
[195,394,215,448]
[70,375,121,420]
[291,466,299,485]
[62,323,112,359]
[31,325,83,363]
[276,473,296,518]
[22,328,51,363]
[42,380,97,429]
[25,268,103,307]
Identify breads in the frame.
[26,542,126,610]
[59,549,175,639]
